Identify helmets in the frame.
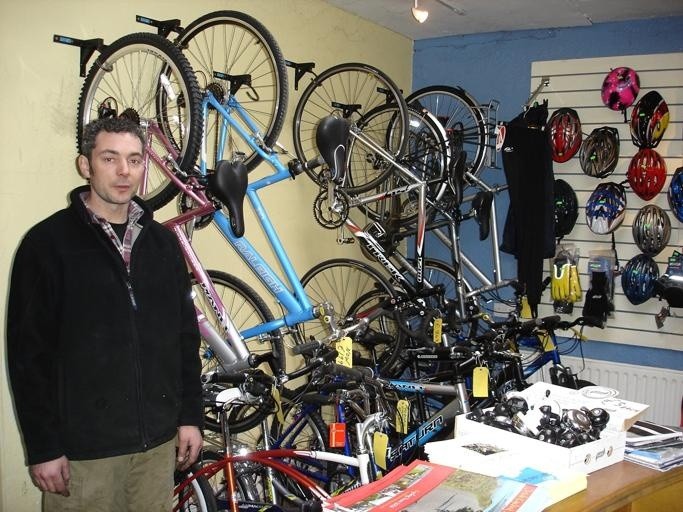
[627,149,667,201]
[578,127,620,178]
[620,254,658,305]
[629,90,669,148]
[584,182,626,235]
[631,203,671,257]
[600,67,640,111]
[666,166,683,223]
[544,107,582,164]
[552,179,578,237]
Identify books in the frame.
[320,419,681,512]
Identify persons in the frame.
[3,116,204,511]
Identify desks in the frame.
[542,461,683,512]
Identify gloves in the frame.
[583,273,613,324]
[550,253,569,302]
[568,264,581,302]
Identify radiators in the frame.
[519,349,683,427]
